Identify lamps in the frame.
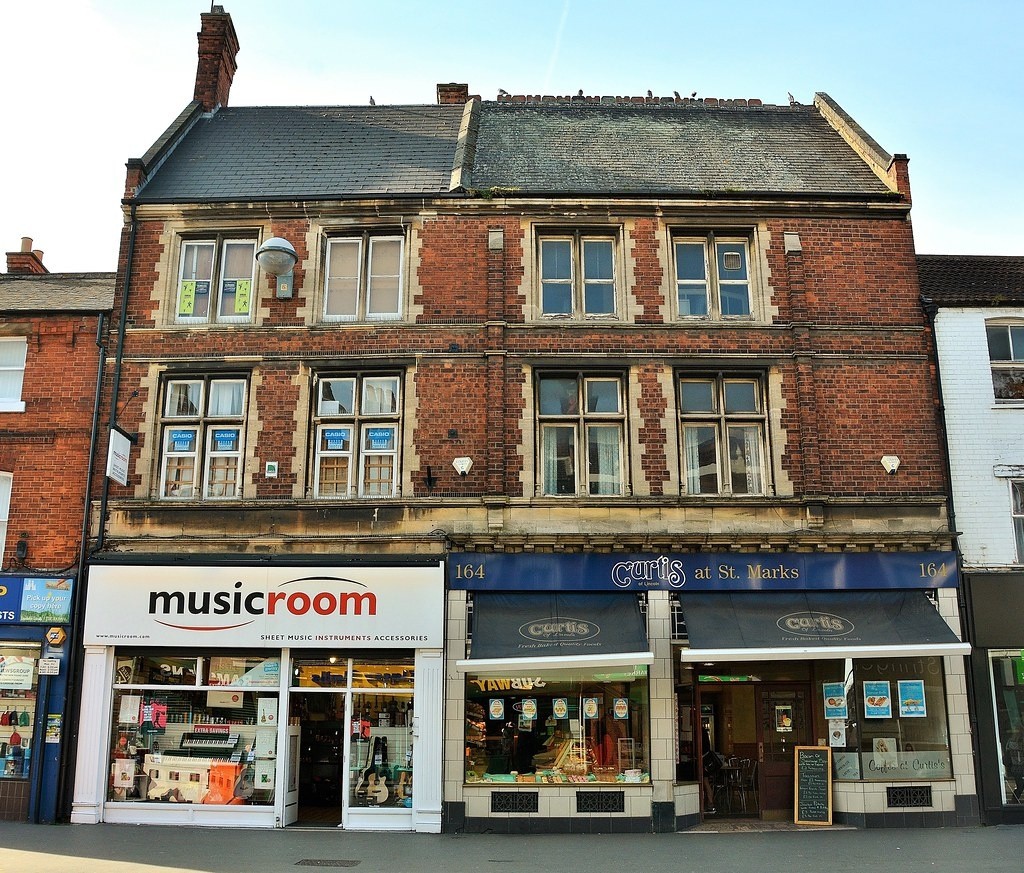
[255,238,295,300]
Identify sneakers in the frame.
[704,807,717,814]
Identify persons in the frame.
[690,707,720,814]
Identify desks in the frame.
[715,766,744,812]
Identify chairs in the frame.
[713,757,758,814]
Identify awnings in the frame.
[681,589,972,662]
[456,591,655,670]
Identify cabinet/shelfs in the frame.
[618,738,642,774]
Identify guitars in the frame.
[397,743,413,801]
[365,742,390,805]
[233,736,257,801]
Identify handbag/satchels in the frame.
[702,751,722,775]
